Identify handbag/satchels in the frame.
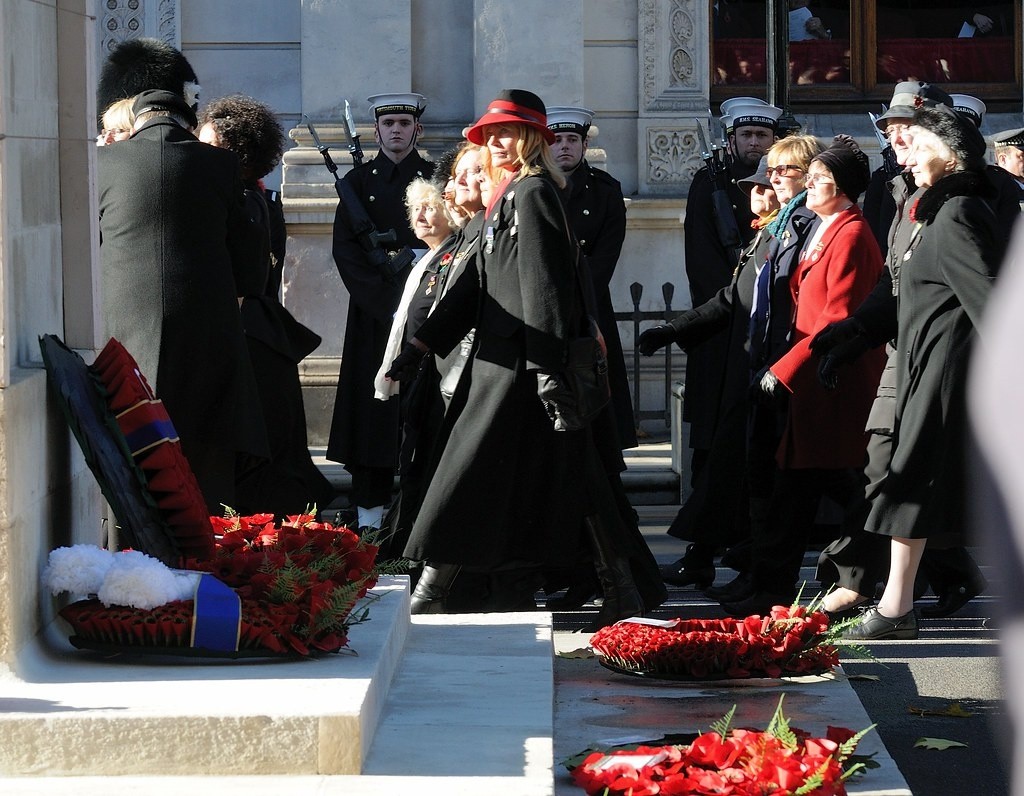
[537,312,611,430]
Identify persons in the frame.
[91,0,1024,639]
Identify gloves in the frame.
[809,316,860,363]
[537,369,580,421]
[385,342,424,381]
[816,351,839,391]
[759,370,780,399]
[636,325,676,357]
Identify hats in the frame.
[991,128,1024,147]
[810,133,871,206]
[367,92,426,120]
[467,88,556,146]
[727,103,783,131]
[720,115,733,133]
[720,97,769,116]
[737,155,772,199]
[875,81,953,132]
[545,104,595,142]
[948,94,986,129]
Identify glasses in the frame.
[804,173,837,184]
[765,164,802,178]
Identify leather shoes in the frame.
[841,606,918,640]
[921,577,984,618]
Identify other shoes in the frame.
[705,565,756,602]
[333,503,358,526]
[358,526,378,544]
[720,591,773,617]
[815,587,874,617]
[410,564,464,613]
[547,568,603,611]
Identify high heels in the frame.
[662,543,716,589]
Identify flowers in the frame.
[34,333,431,664]
[439,251,453,269]
[907,195,921,225]
[588,596,891,694]
[564,700,887,796]
